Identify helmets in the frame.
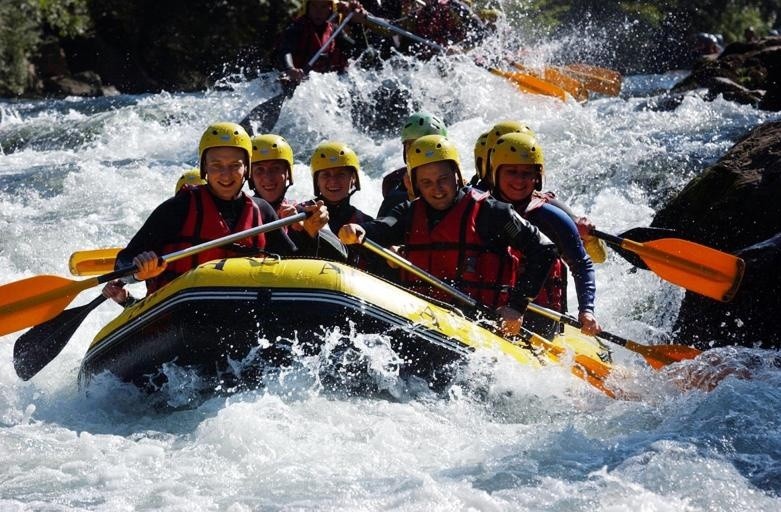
[474,122,545,192]
[198,122,253,180]
[400,113,464,198]
[248,133,293,189]
[312,142,360,197]
[175,169,208,194]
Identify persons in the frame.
[245,134,301,236]
[486,132,601,335]
[102,169,209,309]
[275,0,482,86]
[471,122,535,193]
[337,134,559,343]
[114,122,330,299]
[293,142,376,276]
[376,112,447,270]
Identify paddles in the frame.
[0,199,318,336]
[527,301,702,371]
[13,281,125,381]
[588,227,745,304]
[355,227,620,399]
[241,9,355,135]
[69,248,126,275]
[357,5,625,107]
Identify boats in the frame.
[238,76,410,138]
[76,256,657,425]
